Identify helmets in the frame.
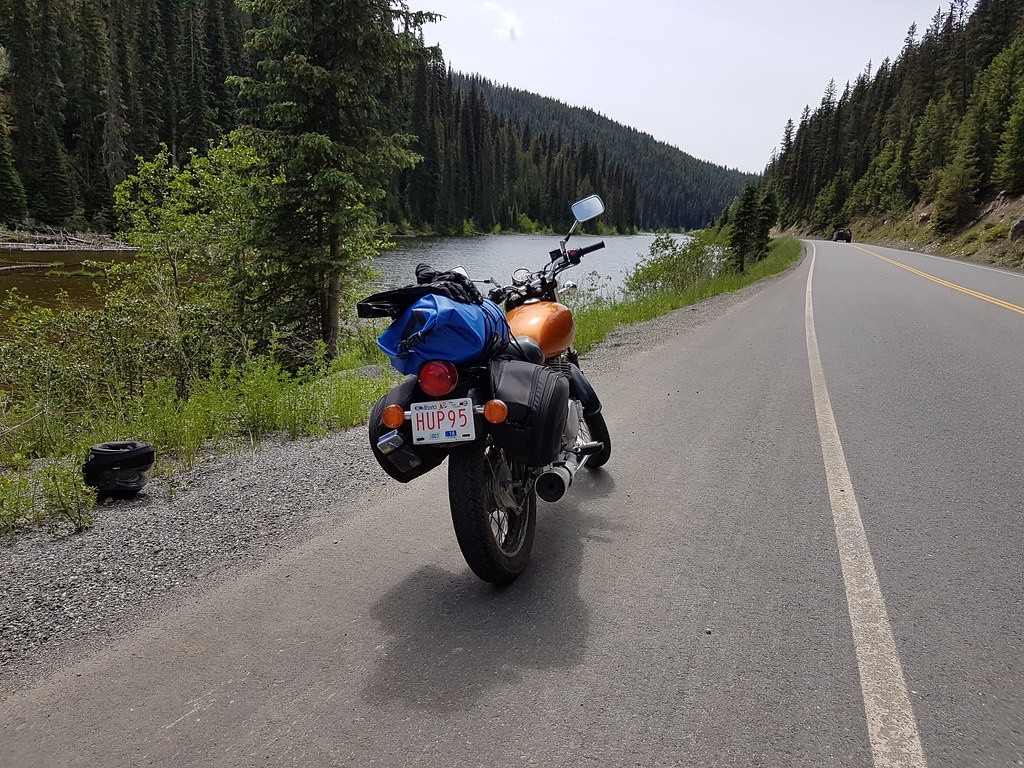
[82,441,155,505]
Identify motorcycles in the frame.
[366,192,611,589]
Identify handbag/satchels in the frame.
[369,378,450,483]
[375,293,510,376]
[482,354,569,467]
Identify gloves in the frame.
[416,262,484,306]
[357,281,472,320]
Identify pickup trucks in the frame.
[833,227,851,243]
